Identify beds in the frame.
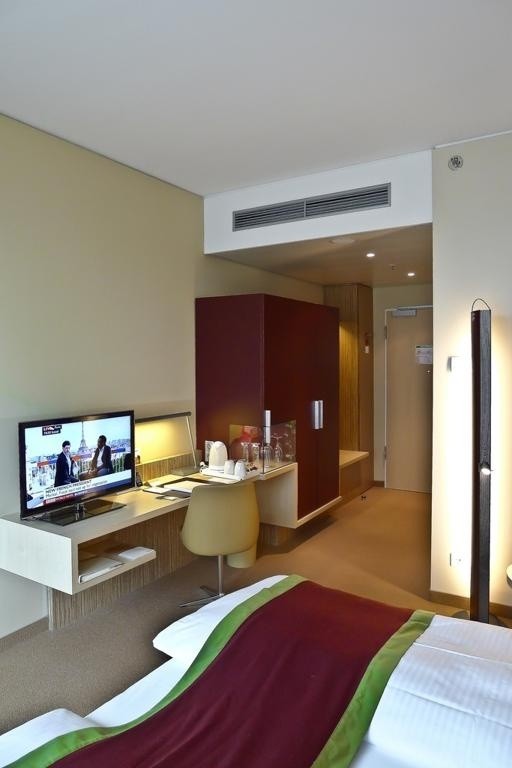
[0,574,512,767]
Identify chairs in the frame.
[179,480,260,609]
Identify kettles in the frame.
[209,441,228,471]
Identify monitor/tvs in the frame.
[18,408,132,526]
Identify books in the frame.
[104,542,154,561]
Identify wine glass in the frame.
[273,436,283,462]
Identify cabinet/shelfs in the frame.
[194,293,339,521]
[323,282,374,487]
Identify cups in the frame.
[224,460,246,478]
[239,441,272,469]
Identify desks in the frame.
[0,461,299,596]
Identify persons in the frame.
[78,435,114,479]
[54,439,78,486]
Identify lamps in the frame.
[135,412,202,476]
